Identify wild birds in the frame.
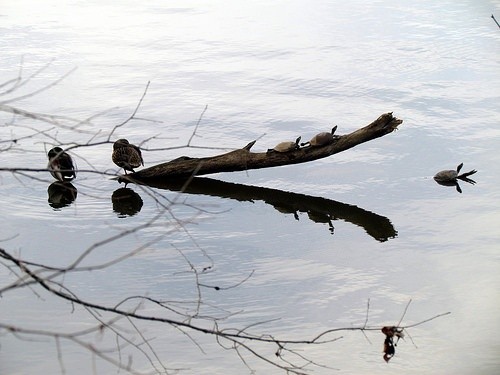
[47,146,76,183]
[111,182,144,220]
[112,139,145,176]
[47,178,78,212]
